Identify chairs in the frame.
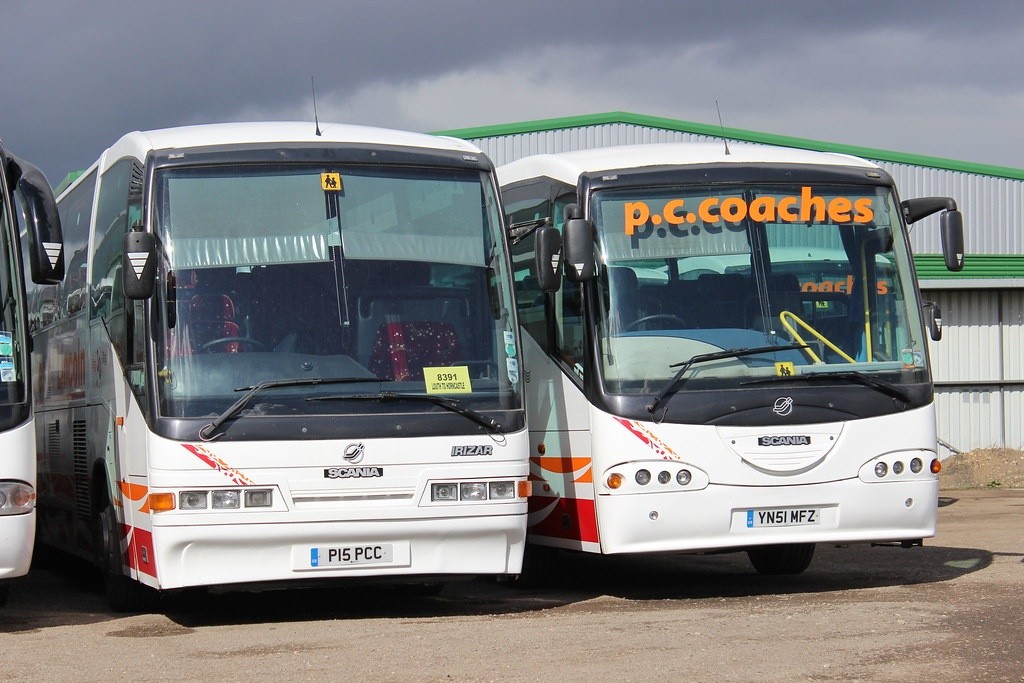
[182,294,239,354]
[369,319,460,382]
[604,266,802,334]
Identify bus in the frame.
[0,141,66,579]
[18,120,563,591]
[432,139,965,578]
